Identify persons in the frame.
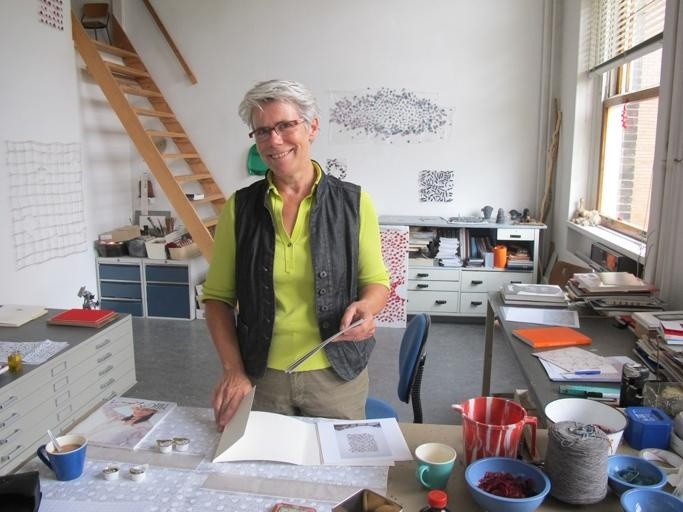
[196,80,390,432]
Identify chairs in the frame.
[80,3,112,45]
[365,313,432,423]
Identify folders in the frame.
[553,380,622,396]
[511,325,591,351]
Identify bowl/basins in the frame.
[601,455,667,499]
[466,456,552,511]
[641,448,682,474]
[671,428,683,457]
[620,489,683,512]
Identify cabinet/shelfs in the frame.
[0,314,138,485]
[460,225,546,324]
[143,254,194,323]
[94,258,142,321]
[408,224,460,325]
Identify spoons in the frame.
[47,430,62,452]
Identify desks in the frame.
[480,289,683,452]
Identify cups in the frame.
[36,435,88,481]
[416,443,456,490]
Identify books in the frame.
[409,227,461,267]
[499,270,660,328]
[506,255,532,270]
[512,327,592,348]
[0,306,49,327]
[630,310,683,382]
[49,309,120,329]
[64,395,178,452]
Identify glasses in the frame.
[249,118,307,139]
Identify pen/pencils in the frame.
[587,392,620,399]
[567,370,603,376]
